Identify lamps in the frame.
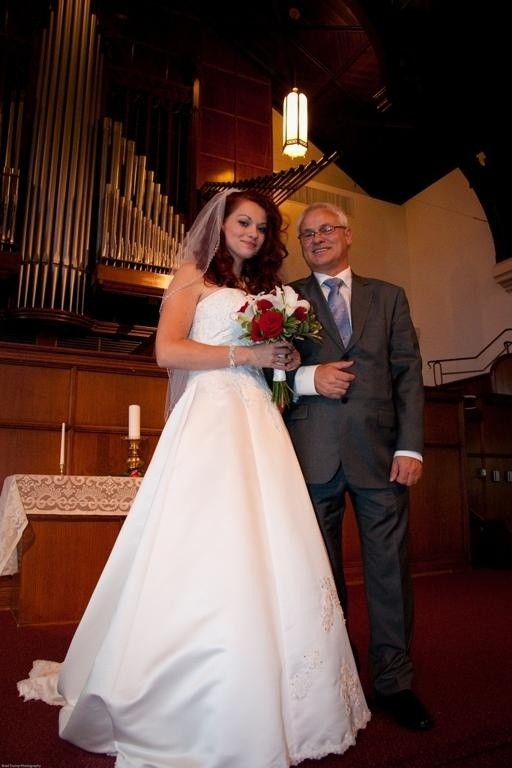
[282,68,309,160]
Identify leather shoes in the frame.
[376,687,434,732]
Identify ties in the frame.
[324,278,352,349]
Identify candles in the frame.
[128,404,141,439]
[60,422,65,464]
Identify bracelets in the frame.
[227,344,239,369]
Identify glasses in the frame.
[297,225,347,240]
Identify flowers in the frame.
[230,284,324,409]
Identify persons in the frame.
[263,200,438,732]
[15,186,372,767]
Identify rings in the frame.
[297,361,302,364]
